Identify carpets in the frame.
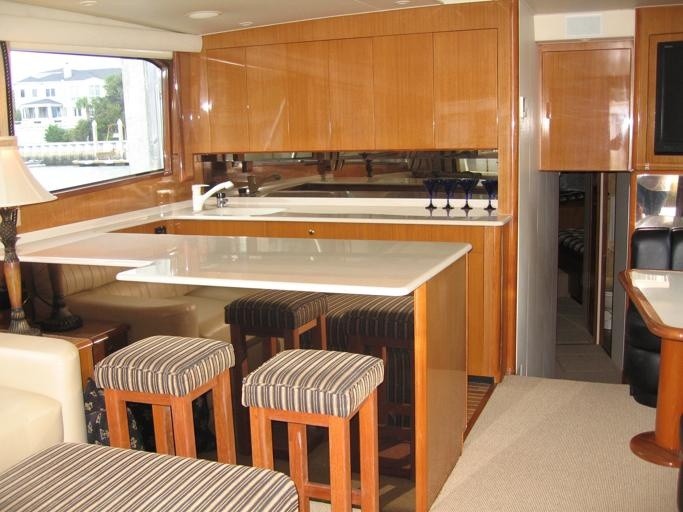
[430,373,683,511]
[463,381,498,444]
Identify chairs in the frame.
[621,227,683,412]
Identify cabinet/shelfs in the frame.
[167,220,485,375]
[539,36,631,173]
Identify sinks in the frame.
[195,208,286,217]
[262,190,351,197]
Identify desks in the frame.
[614,266,682,470]
[2,230,473,512]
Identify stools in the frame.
[322,295,414,484]
[1,441,301,512]
[93,333,239,465]
[222,290,329,464]
[241,346,384,511]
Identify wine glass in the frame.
[443,180,455,209]
[467,175,478,199]
[481,179,497,210]
[422,178,439,209]
[457,179,474,210]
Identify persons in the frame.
[401,151,461,178]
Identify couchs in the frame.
[0,329,90,475]
[30,262,285,438]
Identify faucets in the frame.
[191,180,235,210]
[247,174,281,194]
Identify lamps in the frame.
[0,134,60,336]
